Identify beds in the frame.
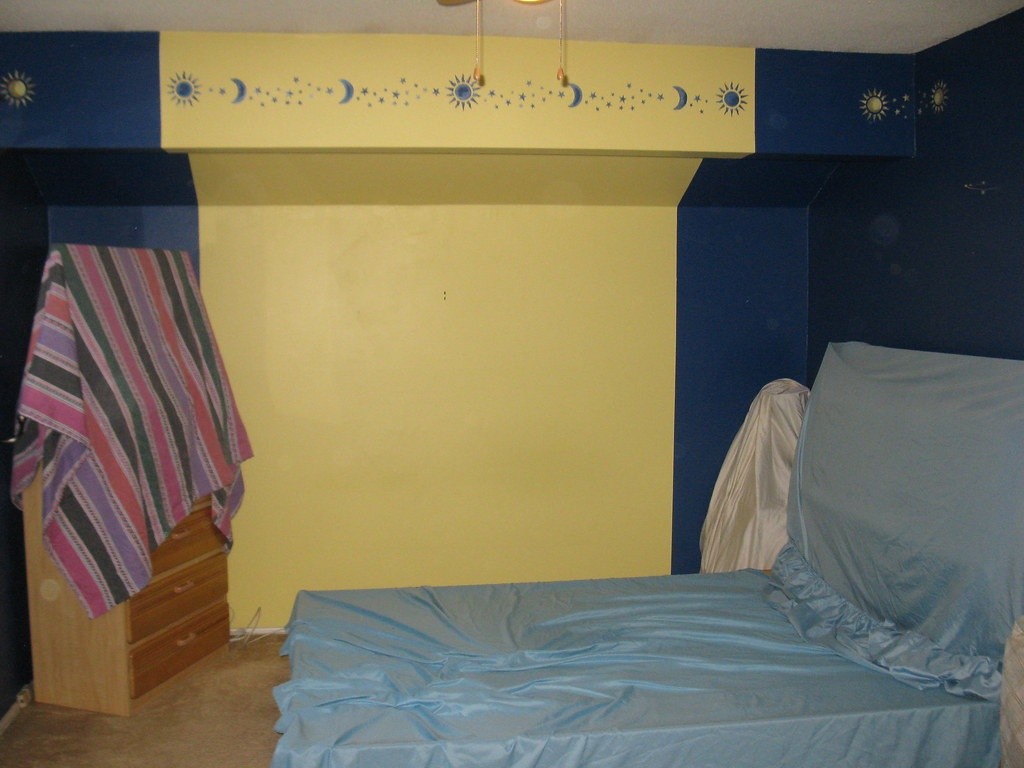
[269,340,1024,768]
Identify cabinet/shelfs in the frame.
[22,462,231,717]
